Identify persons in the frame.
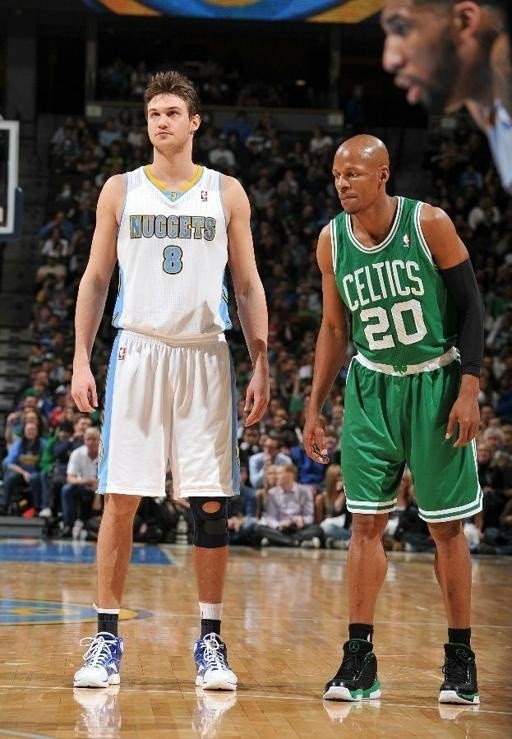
[66,67,275,695]
[68,687,240,738]
[322,700,485,739]
[298,132,494,710]
[0,3,512,563]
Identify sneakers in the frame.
[322,638,382,703]
[261,537,350,550]
[192,631,239,691]
[437,642,481,705]
[39,509,90,539]
[73,630,124,688]
